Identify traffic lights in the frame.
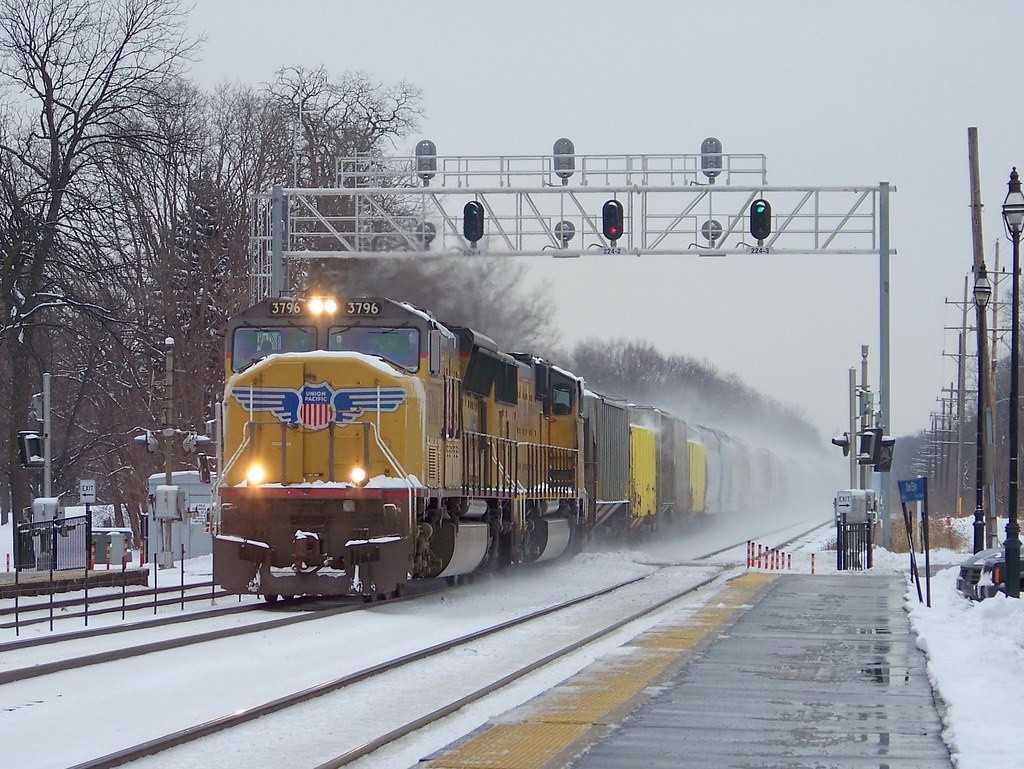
[858,428,880,464]
[874,433,894,473]
[133,431,158,453]
[18,430,45,470]
[196,454,210,484]
[463,201,485,242]
[750,198,772,240]
[181,431,198,455]
[602,199,624,241]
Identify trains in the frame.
[210,295,807,603]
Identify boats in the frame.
[831,431,852,458]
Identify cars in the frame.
[956,544,1024,605]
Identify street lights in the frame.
[974,262,992,555]
[1001,166,1024,601]
